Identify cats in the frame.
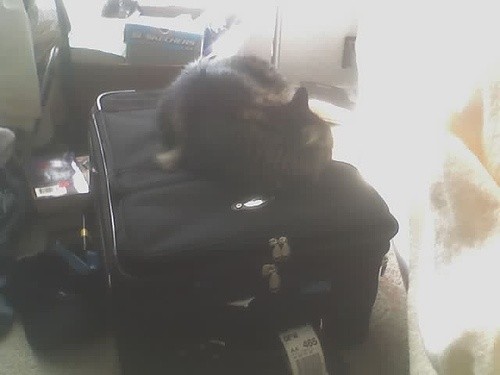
[154,49,334,177]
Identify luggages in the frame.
[87,89,399,373]
